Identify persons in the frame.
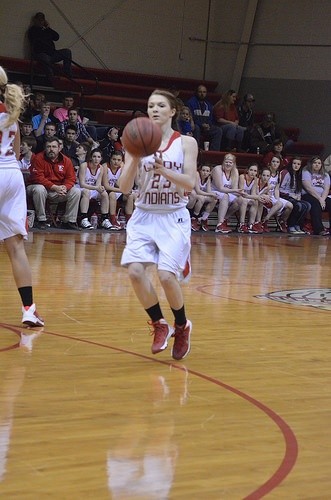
[260,138,293,168]
[28,12,75,81]
[120,90,198,361]
[175,85,292,156]
[186,153,249,233]
[238,155,331,236]
[12,81,133,230]
[0,65,44,327]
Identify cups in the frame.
[204,142,209,151]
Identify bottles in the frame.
[269,188,274,201]
[120,214,125,228]
[91,212,97,229]
[257,146,259,154]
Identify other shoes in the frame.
[38,221,47,230]
[289,226,296,232]
[68,221,83,231]
[111,220,122,229]
[296,225,300,231]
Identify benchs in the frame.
[0,53,327,230]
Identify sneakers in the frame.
[20,327,45,346]
[225,220,232,232]
[169,363,188,401]
[190,218,199,231]
[82,218,94,229]
[171,320,192,359]
[21,303,44,326]
[277,218,287,233]
[198,217,208,231]
[101,219,115,230]
[215,222,229,233]
[147,319,174,354]
[152,376,169,400]
[238,222,269,234]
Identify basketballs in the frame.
[122,117,162,156]
